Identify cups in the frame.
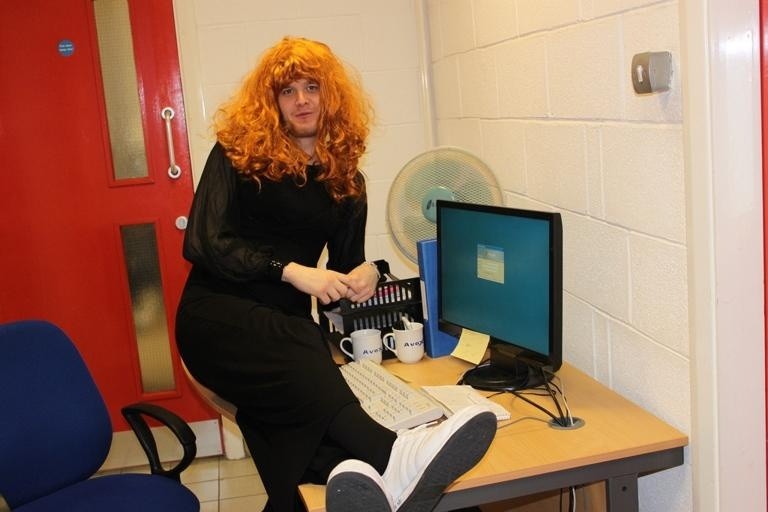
[383,321,425,365]
[339,329,383,365]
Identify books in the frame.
[419,384,511,423]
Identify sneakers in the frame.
[324,458,393,512]
[381,404,498,511]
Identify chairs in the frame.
[0,320,200,512]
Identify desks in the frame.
[180,340,688,512]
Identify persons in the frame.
[174,36,498,512]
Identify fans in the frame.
[386,145,504,266]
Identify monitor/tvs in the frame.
[437,196,564,390]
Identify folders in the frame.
[416,237,460,359]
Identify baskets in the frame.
[318,259,424,366]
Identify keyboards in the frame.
[338,355,443,434]
